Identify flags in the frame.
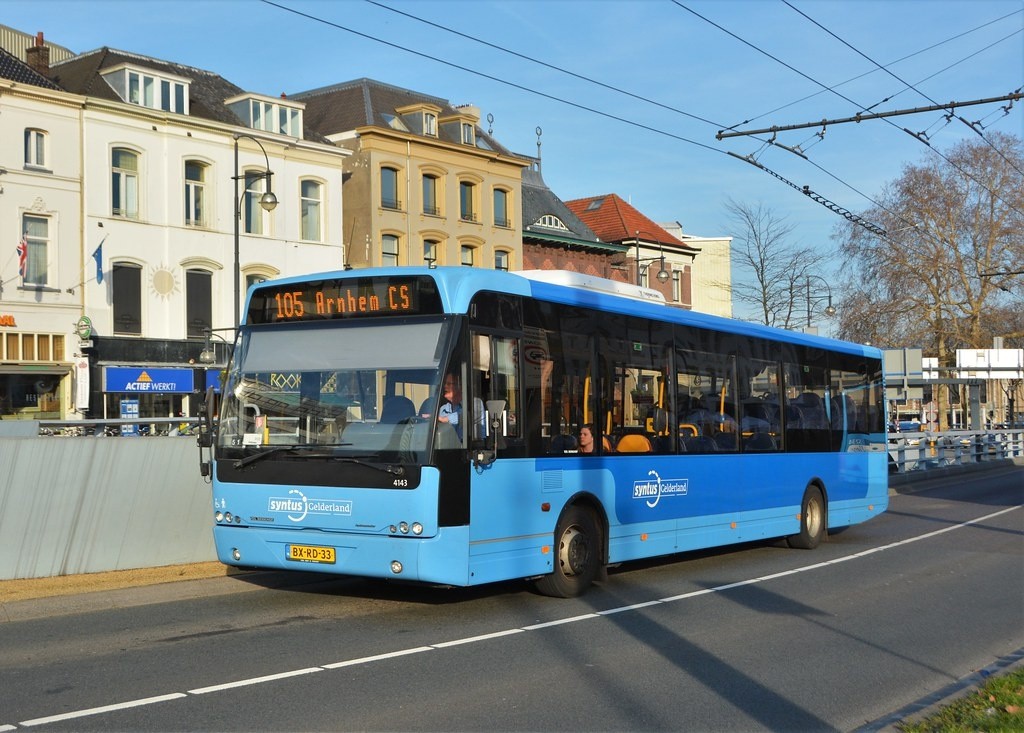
[16,231,28,279]
[93,240,104,284]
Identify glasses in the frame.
[444,381,457,386]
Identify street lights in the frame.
[634,230,670,288]
[199,134,279,364]
[804,273,837,328]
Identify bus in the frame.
[198,265,890,598]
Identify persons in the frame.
[421,371,486,444]
[578,424,598,453]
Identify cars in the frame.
[888,420,921,444]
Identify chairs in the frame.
[380,395,451,423]
[551,392,878,455]
